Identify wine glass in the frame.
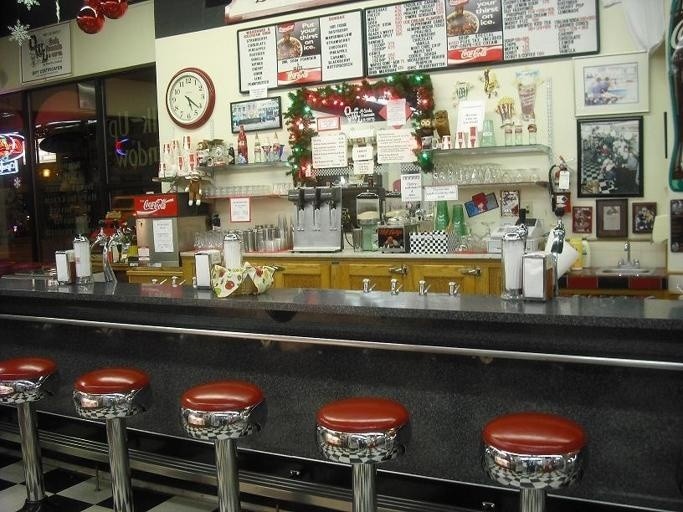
[452,217,496,255]
[430,160,542,183]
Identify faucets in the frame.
[624,241,631,264]
[417,280,433,295]
[361,278,378,293]
[446,280,462,296]
[389,278,404,295]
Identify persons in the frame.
[590,73,611,105]
[592,127,637,182]
[574,210,591,230]
[634,205,654,231]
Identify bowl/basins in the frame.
[228,274,257,295]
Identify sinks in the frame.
[601,268,650,275]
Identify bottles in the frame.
[457,126,476,148]
[503,120,522,146]
[71,234,92,286]
[450,204,467,237]
[478,119,496,146]
[500,233,525,303]
[228,122,280,164]
[434,199,451,231]
[527,118,538,146]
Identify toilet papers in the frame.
[501,232,522,291]
[73,242,93,278]
[522,257,544,299]
[54,254,69,282]
[546,230,577,283]
[223,241,241,270]
[195,255,210,287]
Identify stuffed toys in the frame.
[184,170,202,206]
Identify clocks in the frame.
[165,67,217,132]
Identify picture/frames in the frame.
[595,199,628,237]
[575,117,645,198]
[228,96,284,133]
[572,51,651,116]
[572,206,593,233]
[631,202,658,234]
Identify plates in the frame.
[276,36,303,59]
[447,8,479,33]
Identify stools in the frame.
[312,396,411,511]
[0,355,57,512]
[178,379,267,511]
[70,366,152,512]
[479,410,586,512]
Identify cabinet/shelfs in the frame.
[183,254,267,288]
[400,262,474,294]
[474,264,504,296]
[426,143,552,186]
[331,262,400,291]
[267,259,331,289]
[202,157,291,201]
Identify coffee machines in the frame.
[285,185,386,254]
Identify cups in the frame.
[243,213,291,252]
[497,103,511,125]
[157,134,199,178]
[190,230,224,250]
[441,135,451,150]
[513,68,540,122]
[200,182,292,197]
